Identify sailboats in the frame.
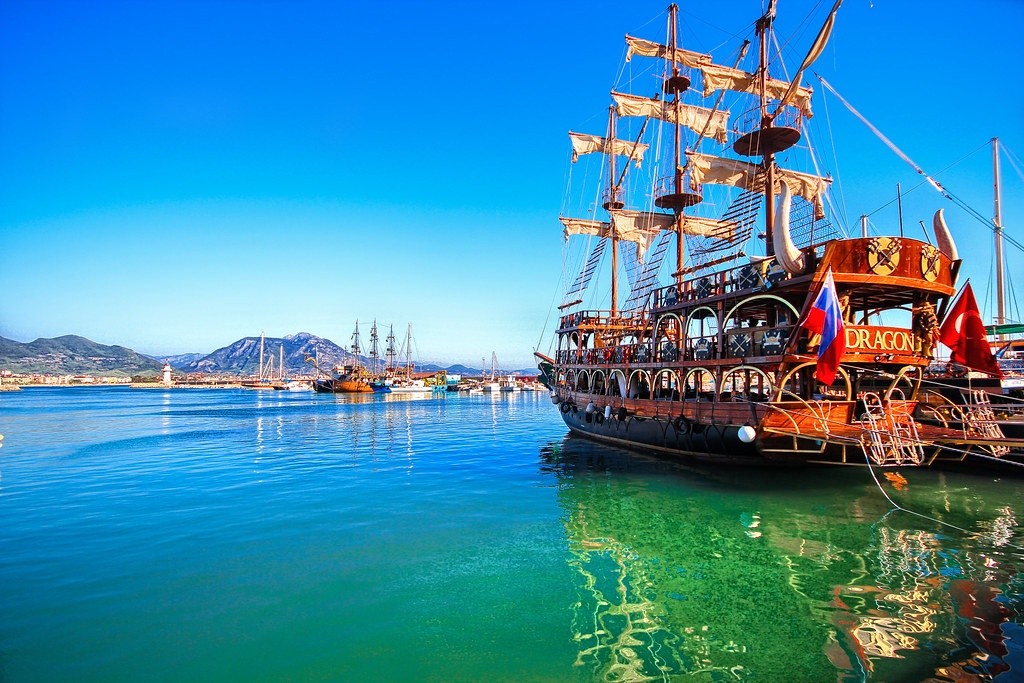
[535,2,1024,485]
[235,318,543,394]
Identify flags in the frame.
[804,273,847,387]
[939,283,1005,381]
